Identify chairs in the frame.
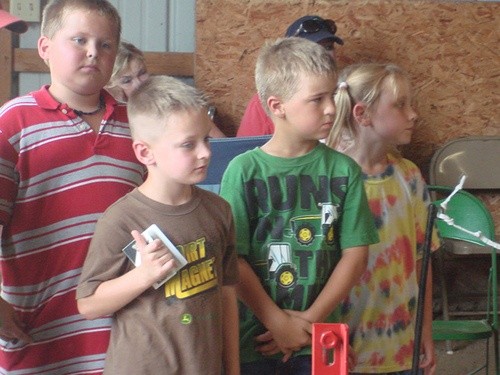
[419,135,500,375]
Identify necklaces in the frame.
[73,94,104,115]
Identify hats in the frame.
[284,16,344,46]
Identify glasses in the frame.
[295,19,337,36]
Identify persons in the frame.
[103,45,228,140]
[237,15,345,139]
[218,37,381,375]
[74,76,241,375]
[1,0,149,375]
[323,61,442,375]
